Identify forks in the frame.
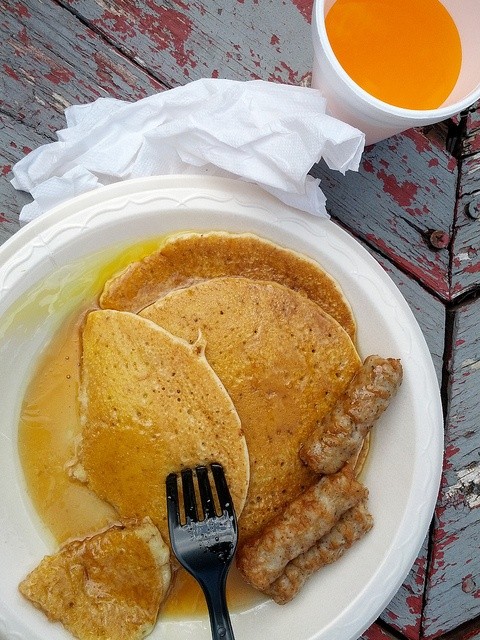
[163,462,240,640]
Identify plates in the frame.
[1,172,447,639]
